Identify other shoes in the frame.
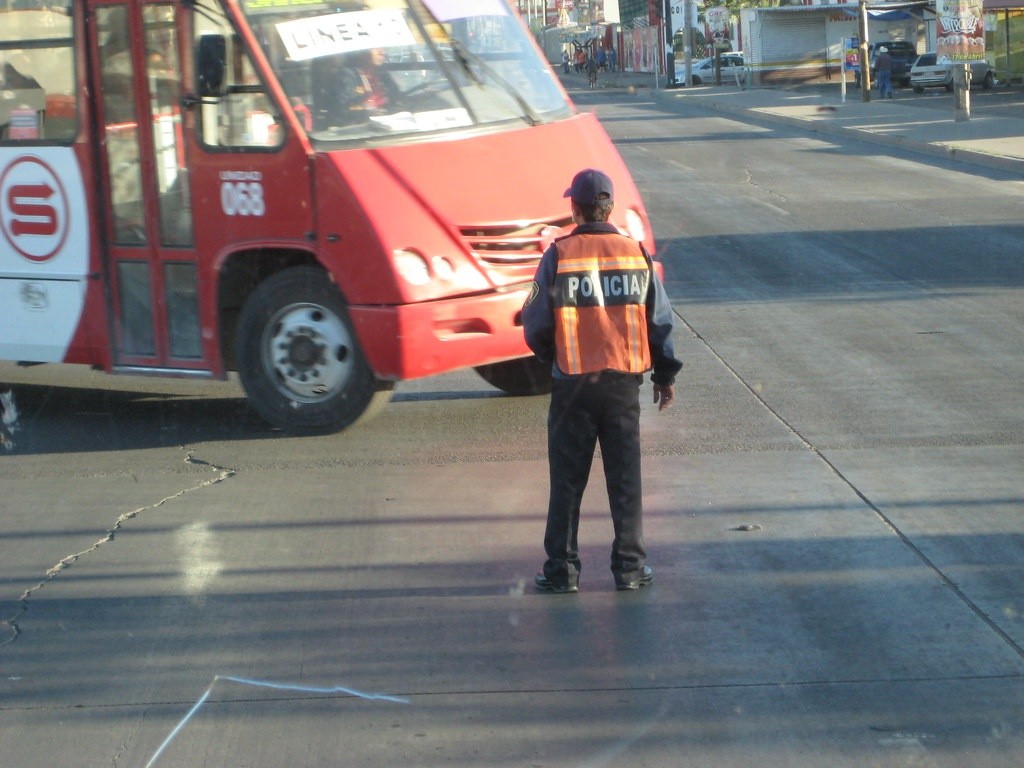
[535,573,579,593]
[617,565,652,591]
[887,92,892,99]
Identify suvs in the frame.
[854,40,918,87]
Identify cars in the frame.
[675,54,745,85]
[721,51,743,57]
[910,51,997,93]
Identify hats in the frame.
[879,46,888,52]
[563,168,614,205]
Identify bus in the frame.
[0,0,668,436]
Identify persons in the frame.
[0,12,302,131]
[563,46,617,85]
[874,46,892,100]
[330,38,401,125]
[527,170,686,593]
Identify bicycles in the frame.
[588,71,598,90]
